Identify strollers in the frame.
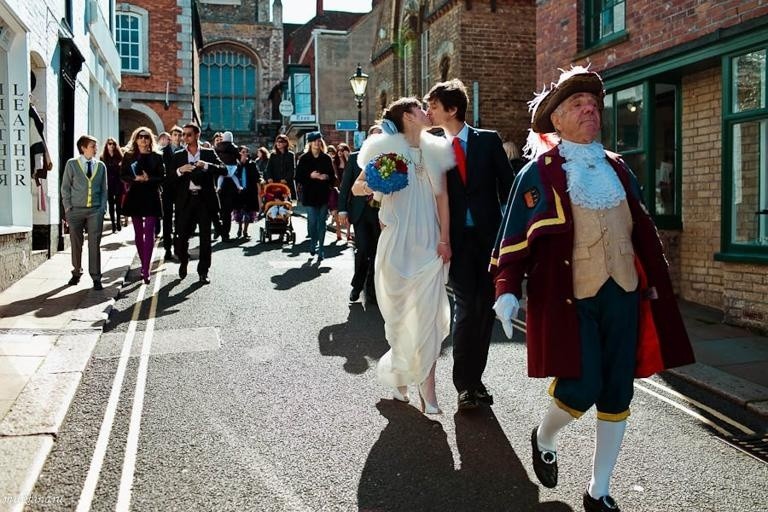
[260,182,297,247]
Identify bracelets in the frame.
[361,180,372,196]
[440,241,450,246]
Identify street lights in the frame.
[349,62,369,132]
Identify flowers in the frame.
[365,152,411,207]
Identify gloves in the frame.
[494,294,520,339]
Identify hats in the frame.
[530,72,603,133]
[221,131,234,142]
[307,132,322,141]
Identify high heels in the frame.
[418,383,441,414]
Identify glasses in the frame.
[136,134,149,139]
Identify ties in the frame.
[85,161,94,179]
[452,135,469,188]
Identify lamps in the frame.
[627,104,636,112]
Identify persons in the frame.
[351,98,459,414]
[423,79,517,408]
[489,62,695,512]
[60,122,523,303]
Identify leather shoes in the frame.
[349,287,363,301]
[582,489,619,511]
[530,425,561,488]
[68,276,81,286]
[200,275,210,284]
[112,222,128,233]
[214,231,251,241]
[93,280,103,290]
[459,392,477,410]
[391,378,410,402]
[477,390,495,408]
[139,267,151,284]
[180,264,187,279]
[309,235,351,260]
